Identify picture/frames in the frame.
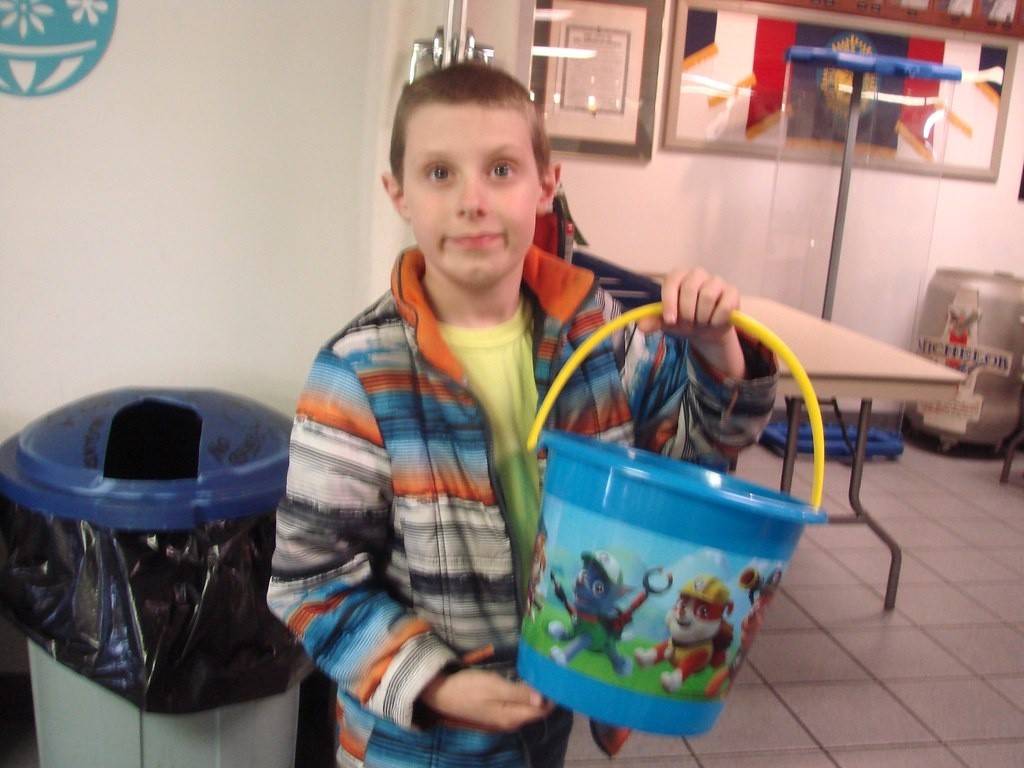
[663,0,1019,184]
[528,0,667,163]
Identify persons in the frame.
[266,55,786,768]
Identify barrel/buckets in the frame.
[514,297,833,738]
[905,265,1024,454]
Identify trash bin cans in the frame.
[0,390,325,768]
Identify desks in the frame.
[650,272,966,612]
